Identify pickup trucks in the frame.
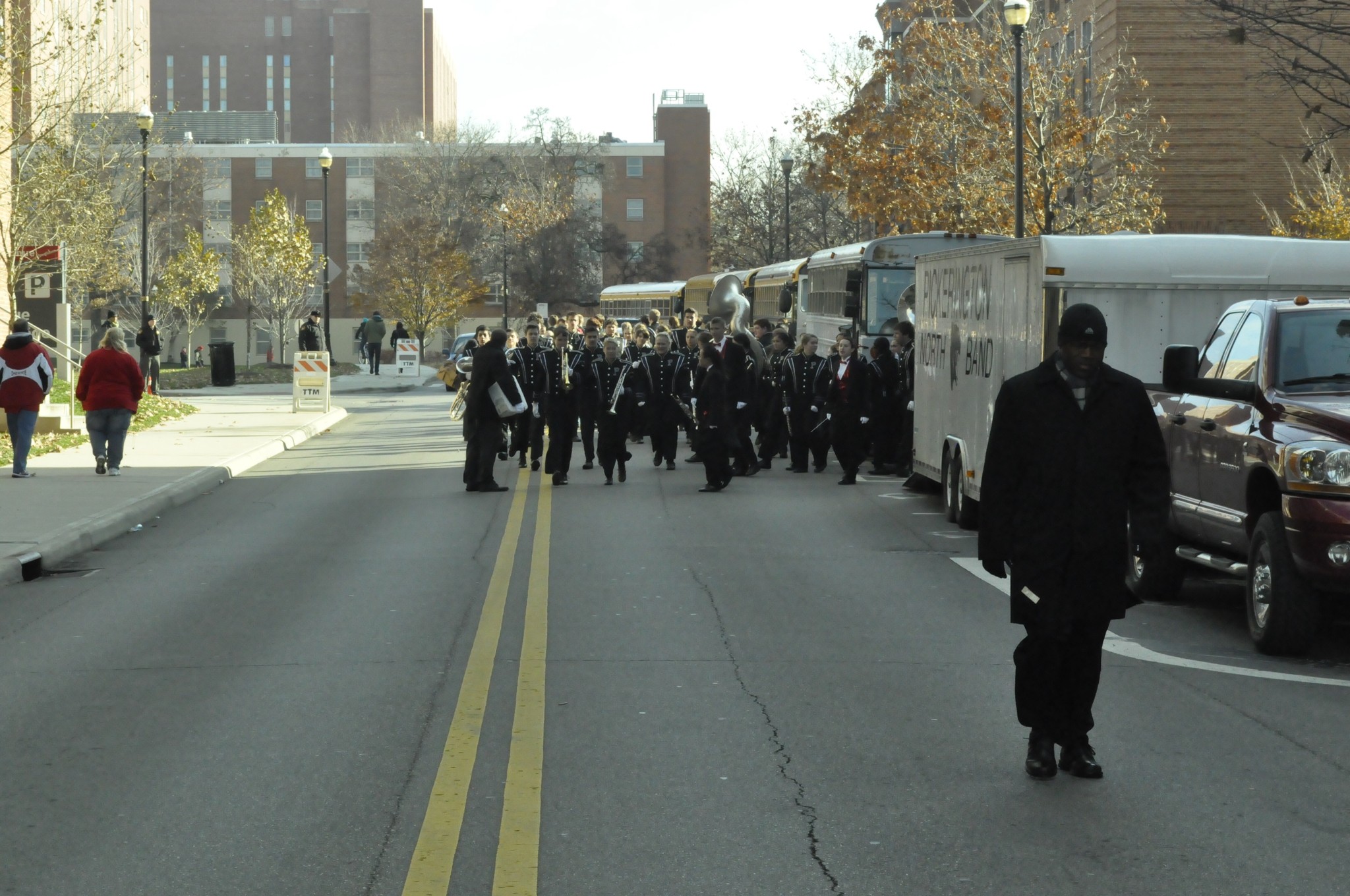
[1117,297,1350,651]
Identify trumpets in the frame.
[671,394,700,425]
[607,365,626,415]
[561,347,574,390]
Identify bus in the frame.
[598,230,1016,372]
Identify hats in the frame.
[13,319,30,333]
[311,310,321,315]
[1057,303,1107,349]
[147,315,156,322]
[107,310,118,319]
[363,318,368,323]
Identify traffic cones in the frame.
[147,376,152,395]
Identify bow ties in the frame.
[839,358,851,365]
[711,342,722,347]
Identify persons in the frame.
[363,311,386,375]
[457,325,491,388]
[101,310,119,329]
[463,329,518,492]
[298,310,328,351]
[497,308,806,493]
[180,347,188,368]
[355,317,368,358]
[75,328,144,476]
[781,333,830,473]
[136,315,164,397]
[267,346,274,362]
[390,322,409,373]
[828,321,913,485]
[0,319,54,478]
[977,303,1171,778]
[194,346,203,368]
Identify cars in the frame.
[441,333,478,391]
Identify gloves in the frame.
[709,426,718,429]
[783,406,832,420]
[465,373,471,380]
[563,368,573,376]
[894,352,899,360]
[637,401,645,406]
[737,402,747,409]
[691,398,697,408]
[531,402,541,418]
[515,402,526,413]
[506,359,517,366]
[632,361,640,369]
[861,417,869,424]
[615,384,624,396]
[907,401,914,411]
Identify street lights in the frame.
[499,203,511,333]
[318,148,339,367]
[1002,0,1034,238]
[135,103,155,332]
[779,154,794,262]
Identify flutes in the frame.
[690,370,696,423]
[784,390,792,436]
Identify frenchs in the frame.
[709,274,764,378]
[897,283,915,329]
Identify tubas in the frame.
[449,357,474,421]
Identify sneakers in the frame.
[96,456,124,476]
[13,469,32,477]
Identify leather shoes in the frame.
[1058,741,1103,779]
[1025,729,1057,778]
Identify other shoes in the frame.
[369,366,380,375]
[462,430,914,493]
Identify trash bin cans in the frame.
[207,341,236,386]
